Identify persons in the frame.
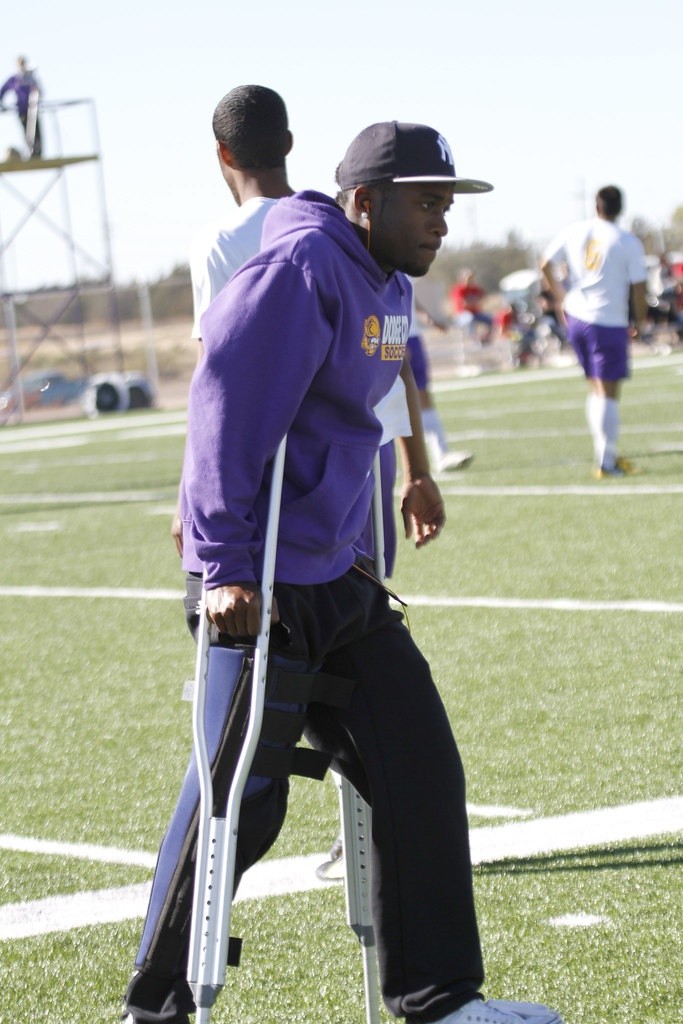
[0,55,43,161]
[541,185,648,481]
[117,84,683,1024]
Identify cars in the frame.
[0,370,89,411]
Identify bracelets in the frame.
[403,470,430,482]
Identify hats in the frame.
[335,120,494,194]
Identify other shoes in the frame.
[437,450,474,471]
[597,465,627,484]
[617,458,640,476]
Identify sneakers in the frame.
[430,997,563,1024]
